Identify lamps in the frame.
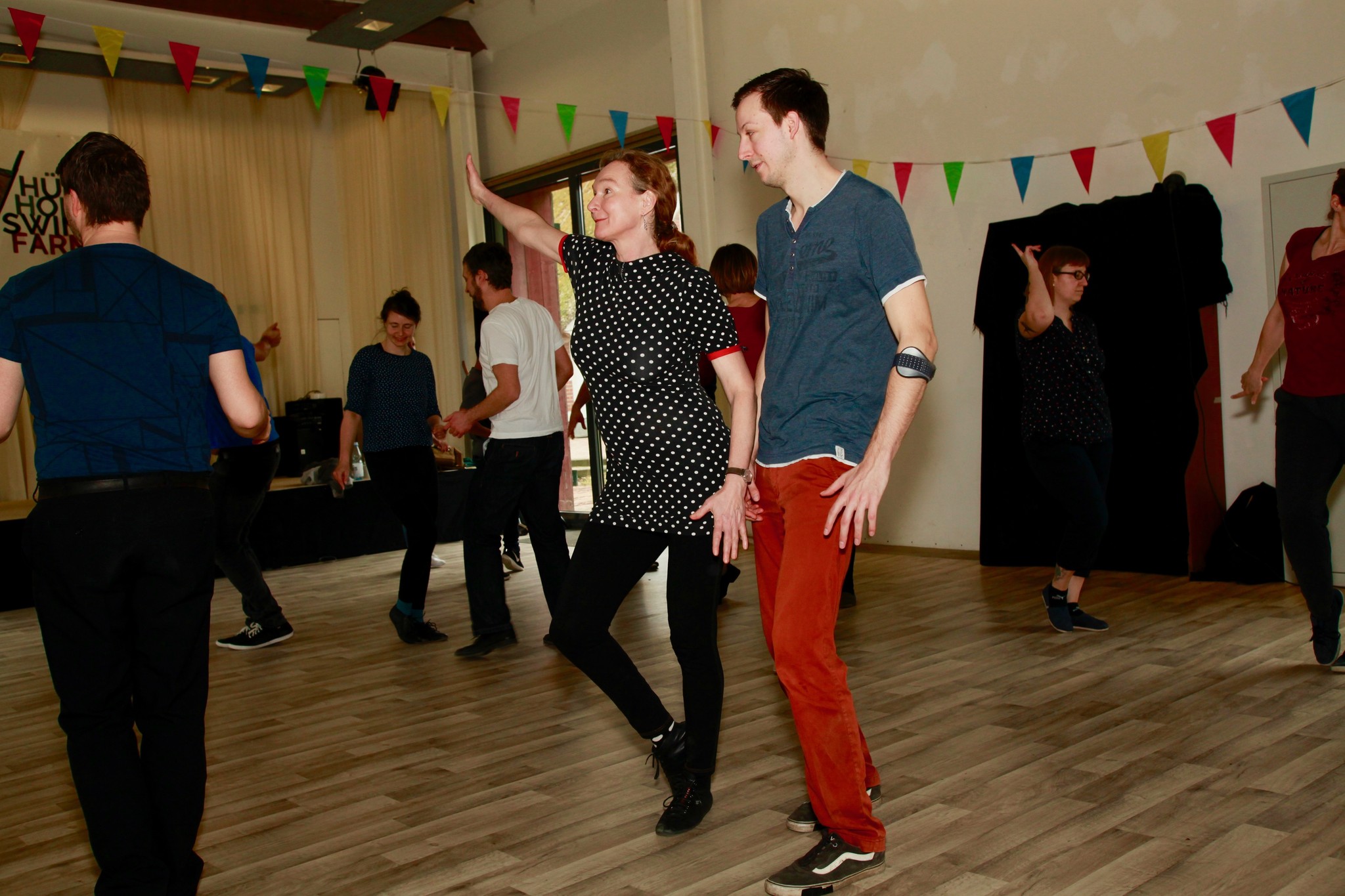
[354,67,400,111]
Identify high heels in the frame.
[717,563,740,605]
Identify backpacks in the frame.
[1190,480,1283,586]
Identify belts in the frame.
[38,476,210,497]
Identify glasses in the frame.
[1051,270,1090,282]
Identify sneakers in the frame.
[417,619,448,641]
[214,619,294,649]
[764,826,886,896]
[1309,586,1344,666]
[454,626,517,656]
[389,606,421,645]
[786,785,883,834]
[645,721,686,796]
[655,776,712,837]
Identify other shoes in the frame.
[1041,583,1072,633]
[502,546,524,573]
[1069,612,1109,632]
[502,572,510,581]
[839,591,856,608]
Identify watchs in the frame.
[725,467,753,483]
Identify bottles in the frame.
[352,442,364,480]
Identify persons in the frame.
[1230,168,1345,672]
[1012,243,1110,632]
[0,67,938,896]
